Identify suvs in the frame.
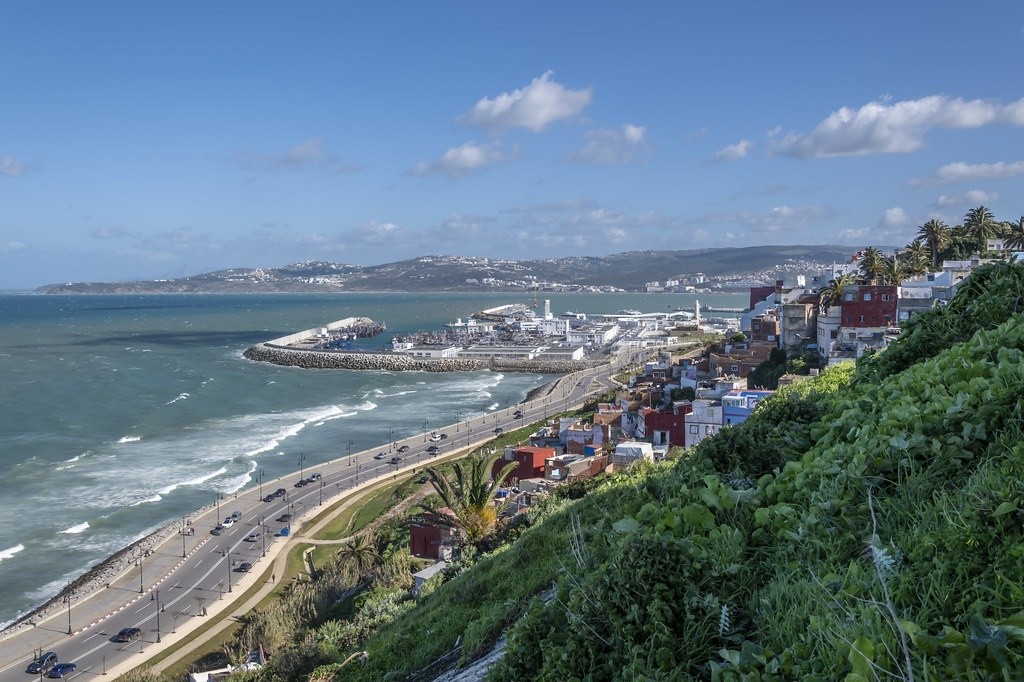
[221,511,242,529]
[117,627,141,642]
[391,457,402,464]
[398,445,409,452]
[376,452,387,459]
[25,652,59,674]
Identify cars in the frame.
[495,428,503,433]
[249,533,262,542]
[514,411,523,420]
[47,663,77,678]
[426,433,448,456]
[263,472,321,502]
[280,514,292,522]
[239,563,252,572]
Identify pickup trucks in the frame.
[213,526,226,535]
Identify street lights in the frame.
[422,418,430,443]
[134,490,234,644]
[454,402,501,446]
[344,429,401,487]
[62,580,73,634]
[33,645,43,682]
[255,467,266,502]
[257,516,270,558]
[282,491,295,515]
[298,452,306,480]
